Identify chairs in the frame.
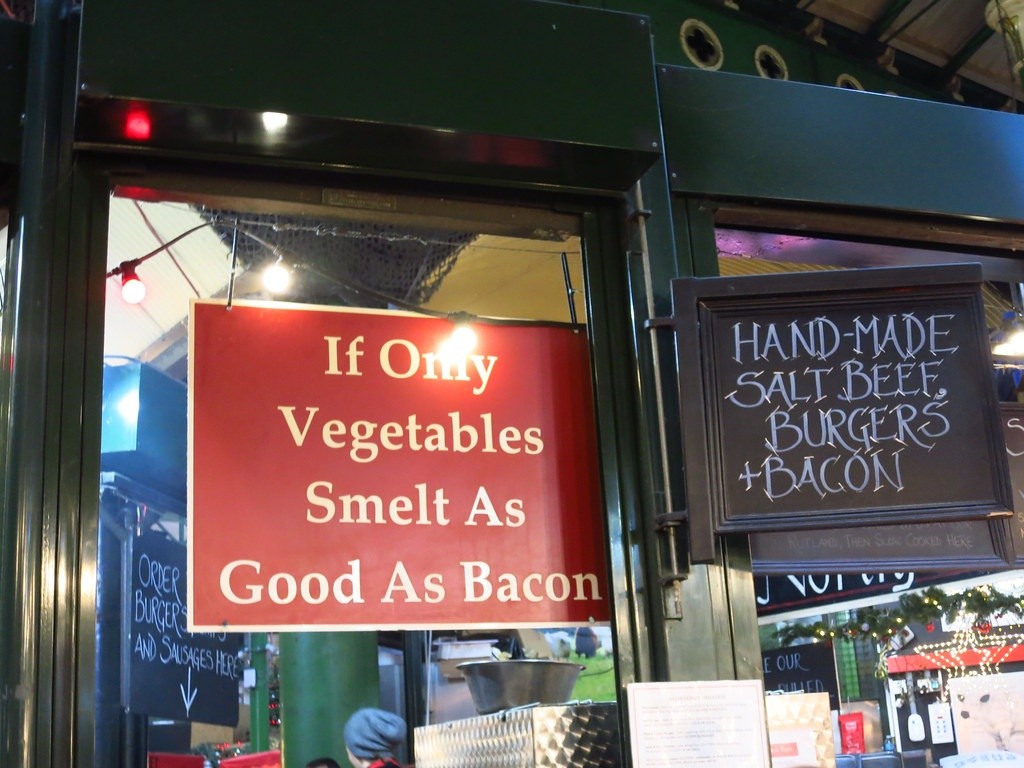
[833,747,942,768]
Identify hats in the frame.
[344,708,409,760]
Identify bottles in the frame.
[885,736,894,752]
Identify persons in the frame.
[340,708,408,768]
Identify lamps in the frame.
[114,257,146,304]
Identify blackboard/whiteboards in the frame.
[131,534,239,728]
[763,643,841,711]
[700,286,1016,533]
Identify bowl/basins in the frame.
[456,660,586,714]
